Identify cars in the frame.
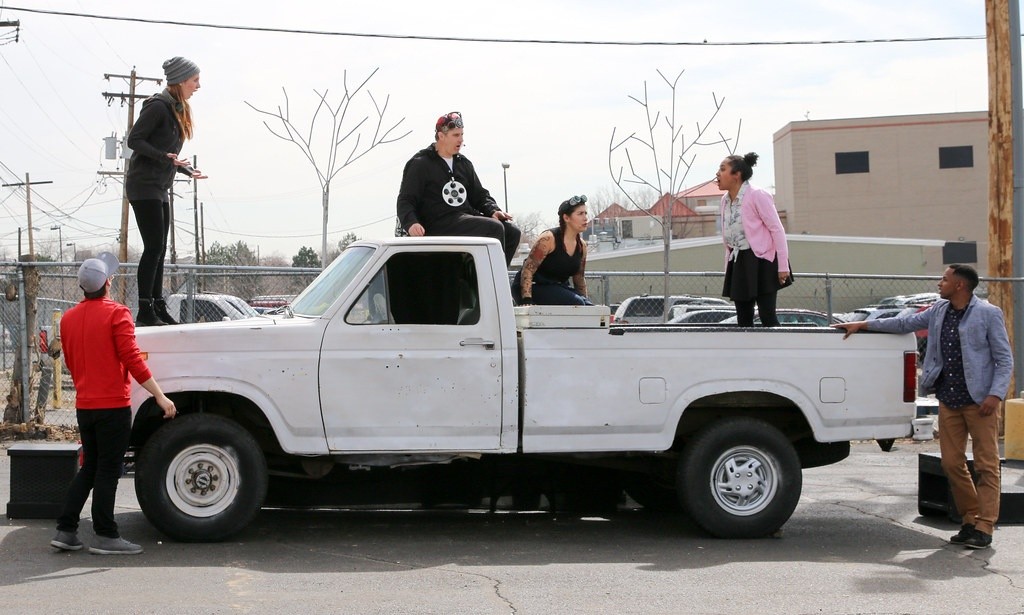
[611,292,942,365]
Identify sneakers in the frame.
[50,531,83,550]
[964,530,992,549]
[88,534,142,554]
[951,523,975,544]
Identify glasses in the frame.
[109,275,115,280]
[569,195,587,206]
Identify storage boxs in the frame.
[514,303,611,328]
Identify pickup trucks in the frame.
[123,238,921,543]
[36,294,296,375]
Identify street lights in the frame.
[502,162,510,213]
[50,225,64,300]
[66,242,77,275]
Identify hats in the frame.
[162,57,201,85]
[436,112,463,131]
[78,251,119,293]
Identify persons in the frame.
[51,252,177,554]
[397,116,521,267]
[830,264,1013,548]
[511,198,593,306]
[717,152,795,327]
[126,57,208,327]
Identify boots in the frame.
[135,299,168,326]
[154,298,180,324]
